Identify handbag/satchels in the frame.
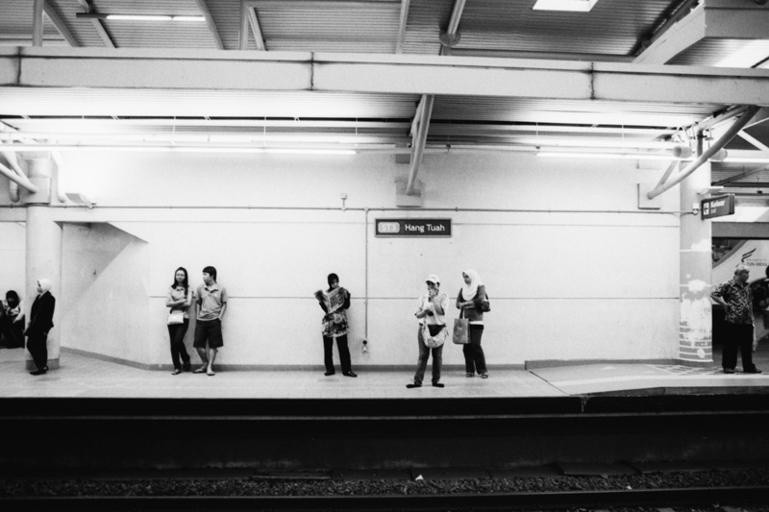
[167,312,184,325]
[453,319,471,344]
[421,326,447,347]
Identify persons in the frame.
[23,279,55,375]
[2,290,25,349]
[317,273,358,377]
[456,269,491,378]
[193,267,228,376]
[406,276,448,388]
[709,266,762,373]
[751,266,768,353]
[165,267,194,375]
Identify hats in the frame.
[426,275,440,286]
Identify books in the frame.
[315,286,344,315]
